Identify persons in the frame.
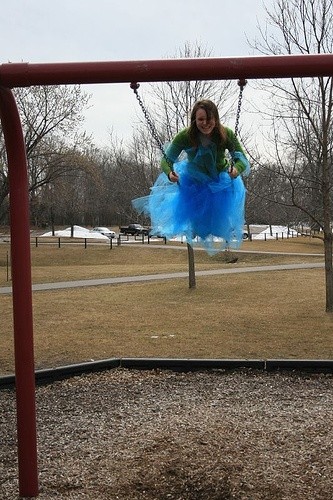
[131,101,251,254]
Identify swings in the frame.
[126,79,252,199]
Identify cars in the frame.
[93,227,116,238]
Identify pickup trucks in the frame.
[120,223,149,236]
